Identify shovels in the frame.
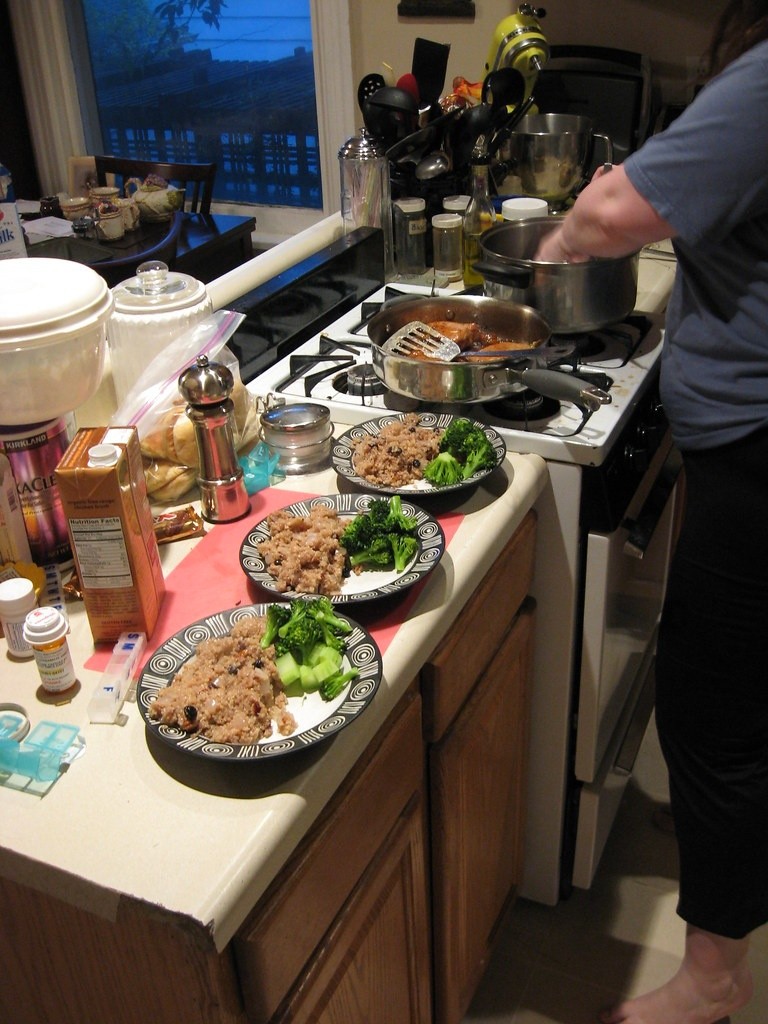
[384,125,432,165]
[412,38,451,109]
[382,321,575,361]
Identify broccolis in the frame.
[260,595,360,701]
[339,496,420,572]
[448,367,472,400]
[424,418,497,485]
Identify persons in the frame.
[532,38,768,1024]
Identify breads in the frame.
[139,380,249,504]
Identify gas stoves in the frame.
[213,226,666,466]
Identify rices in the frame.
[351,412,446,488]
[258,505,363,595]
[146,615,298,744]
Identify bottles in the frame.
[393,198,426,276]
[74,215,96,239]
[0,578,35,658]
[502,198,548,222]
[338,128,390,226]
[23,607,76,692]
[107,261,213,409]
[444,146,497,285]
[432,214,463,282]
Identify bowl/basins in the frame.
[90,187,120,207]
[0,258,115,425]
[60,198,91,220]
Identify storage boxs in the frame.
[53,425,164,644]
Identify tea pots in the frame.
[125,175,183,222]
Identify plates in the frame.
[331,413,507,493]
[137,603,383,760]
[239,494,445,603]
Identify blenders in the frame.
[484,5,612,209]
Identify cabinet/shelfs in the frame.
[1,509,537,1024]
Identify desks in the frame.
[15,190,258,288]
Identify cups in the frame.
[96,199,139,239]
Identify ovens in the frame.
[519,354,687,909]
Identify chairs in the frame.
[102,156,217,213]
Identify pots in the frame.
[471,216,643,333]
[367,295,611,405]
